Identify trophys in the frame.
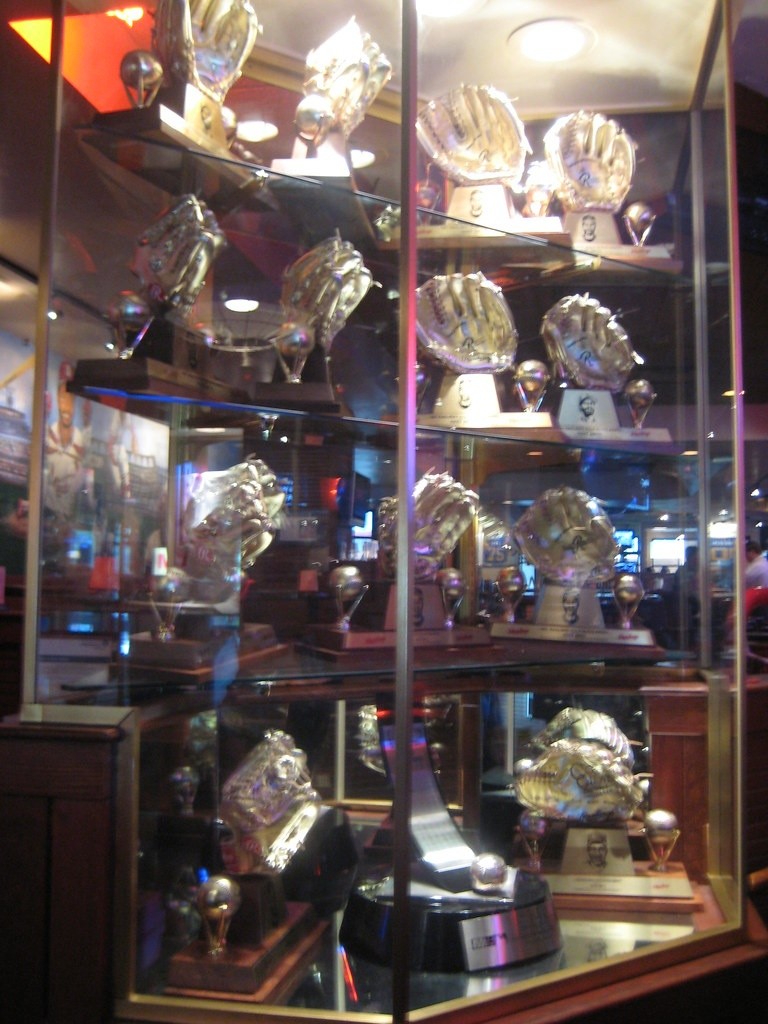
[125,0,706,1004]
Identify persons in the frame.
[0,483,57,608]
[674,545,700,595]
[746,541,767,589]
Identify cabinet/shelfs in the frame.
[0,1,768,1024]
